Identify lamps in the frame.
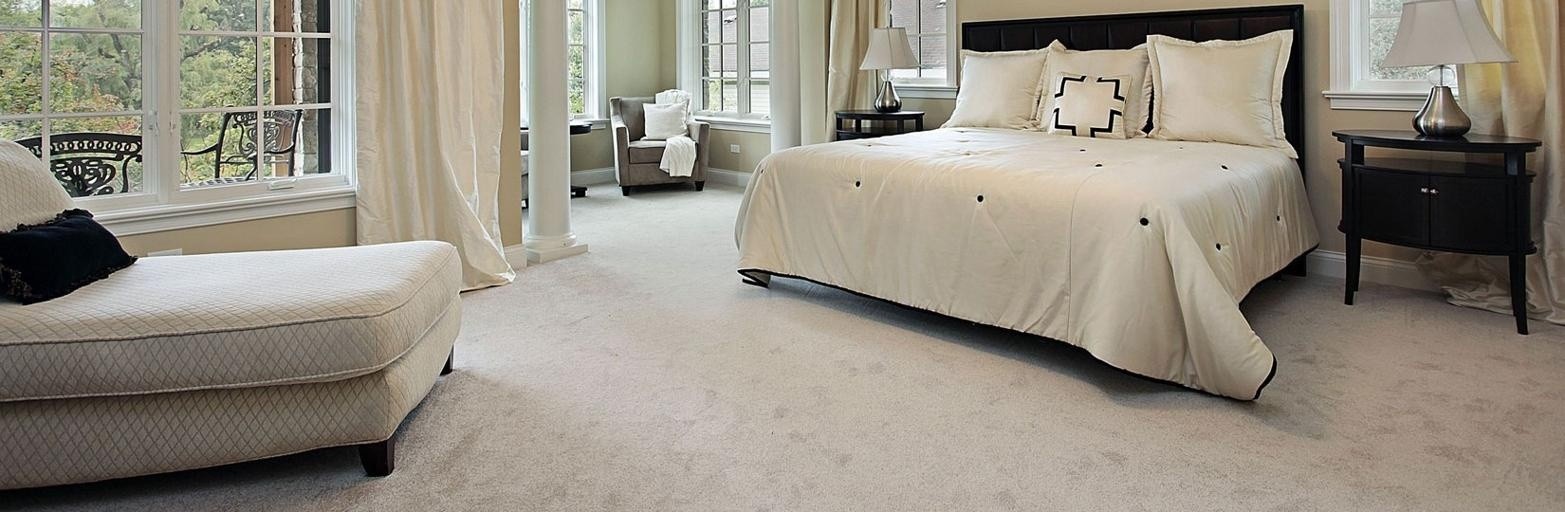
[857,25,922,112]
[1377,1,1518,136]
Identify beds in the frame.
[728,3,1311,405]
[0,138,464,479]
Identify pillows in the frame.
[642,102,690,141]
[1034,40,1158,142]
[0,203,138,304]
[936,49,1052,130]
[1048,72,1136,140]
[1144,28,1302,157]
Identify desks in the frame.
[567,123,597,200]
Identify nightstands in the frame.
[835,109,925,144]
[1332,122,1541,335]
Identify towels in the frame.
[659,136,699,177]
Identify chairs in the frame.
[180,102,304,190]
[1,131,146,196]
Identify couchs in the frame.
[607,93,710,196]
